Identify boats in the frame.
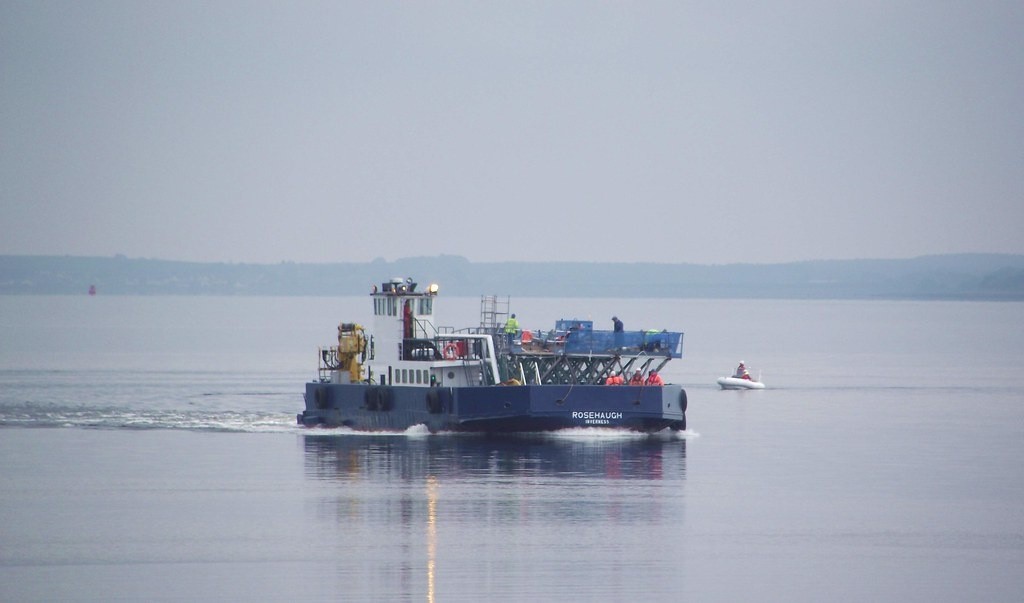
[293,292,687,436]
[717,377,766,389]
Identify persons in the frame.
[627,368,644,386]
[606,371,623,386]
[645,369,664,386]
[505,314,518,345]
[737,361,753,381]
[612,317,623,333]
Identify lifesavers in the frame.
[441,342,460,362]
[313,385,329,410]
[424,388,438,416]
[361,387,391,411]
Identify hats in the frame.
[610,370,617,377]
[635,367,642,371]
[650,369,657,372]
